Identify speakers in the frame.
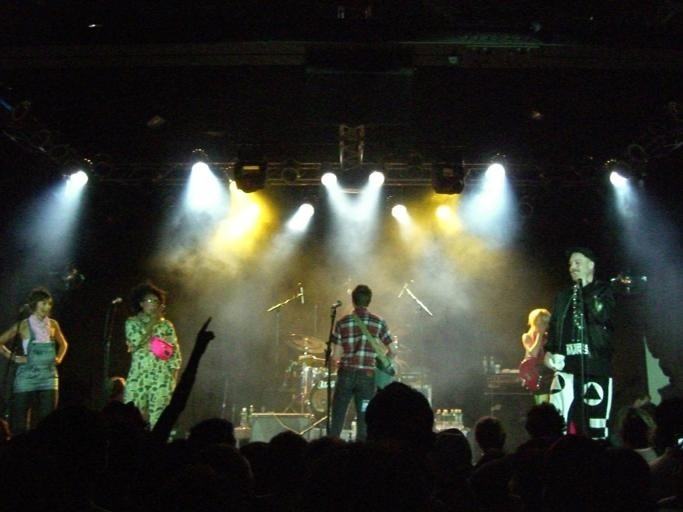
[250,412,312,444]
[461,372,536,430]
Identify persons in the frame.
[122,281,184,428]
[518,307,553,406]
[544,246,618,440]
[0,286,69,432]
[0,375,683,512]
[324,283,398,446]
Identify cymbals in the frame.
[281,334,327,354]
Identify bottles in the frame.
[240,404,255,427]
[434,408,463,431]
[482,355,495,374]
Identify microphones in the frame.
[331,300,342,308]
[111,297,122,305]
[17,304,29,315]
[298,282,305,304]
[397,280,409,298]
[576,275,584,292]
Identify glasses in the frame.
[145,299,158,303]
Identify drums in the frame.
[309,377,354,425]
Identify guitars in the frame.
[300,356,399,376]
[520,356,553,393]
